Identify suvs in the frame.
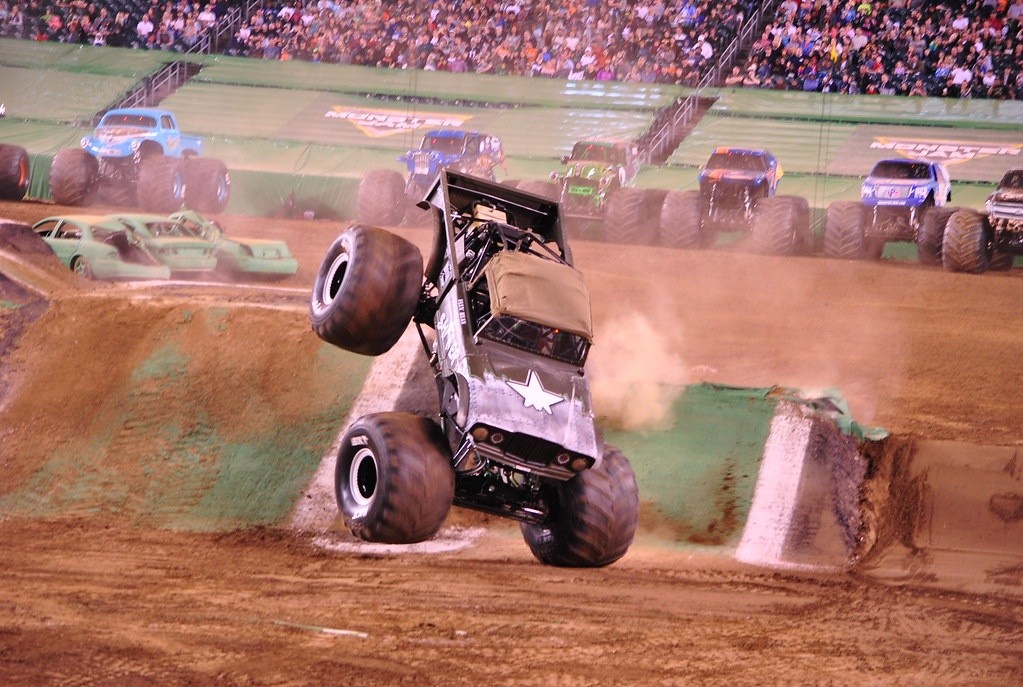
[658,147,813,257]
[356,129,514,228]
[514,143,673,245]
[308,168,643,566]
[940,168,1023,275]
[820,158,960,266]
[50,108,232,215]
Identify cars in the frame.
[166,211,298,285]
[31,216,170,282]
[109,214,218,278]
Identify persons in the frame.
[0,0,1023,100]
[284,190,295,219]
